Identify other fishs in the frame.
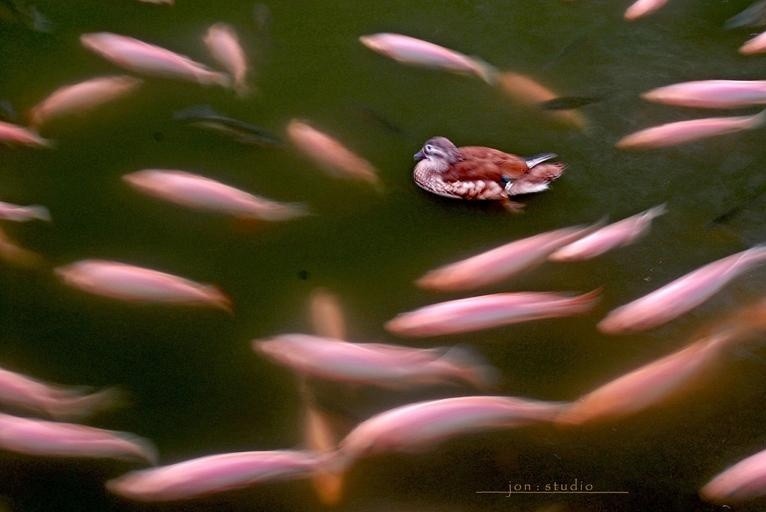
[0,0,766,512]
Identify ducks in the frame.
[412,136,566,215]
